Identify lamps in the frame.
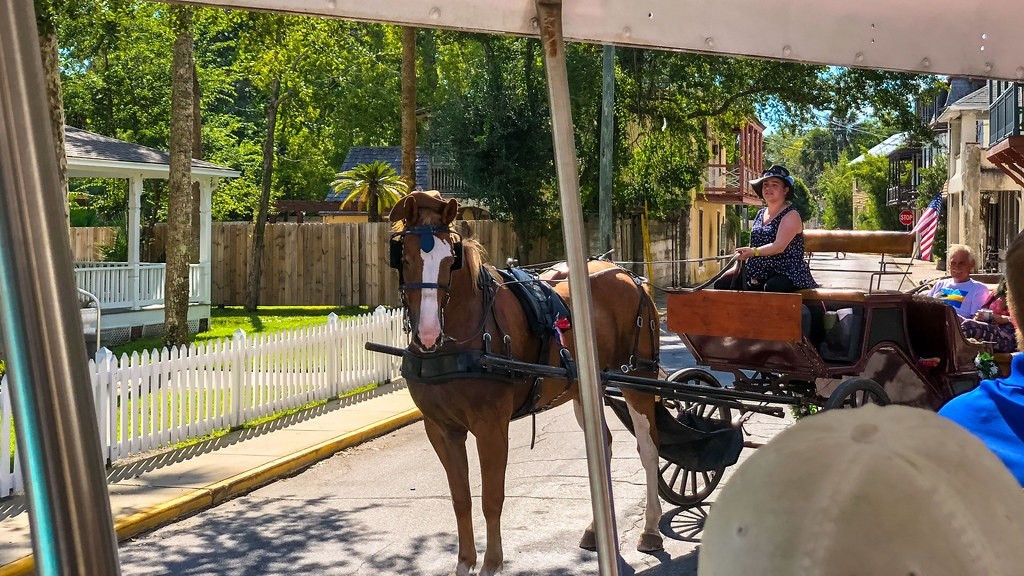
[712,143,719,156]
[898,160,908,176]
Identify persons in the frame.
[729,163,822,291]
[960,277,1017,353]
[936,229,1024,490]
[927,242,989,319]
[835,228,846,259]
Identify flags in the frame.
[910,192,942,258]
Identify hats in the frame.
[748,165,798,202]
[697,403,1024,576]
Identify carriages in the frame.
[361,187,1019,576]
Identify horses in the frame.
[397,193,665,575]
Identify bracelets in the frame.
[753,247,759,256]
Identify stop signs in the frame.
[899,210,915,225]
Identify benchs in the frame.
[792,228,916,305]
